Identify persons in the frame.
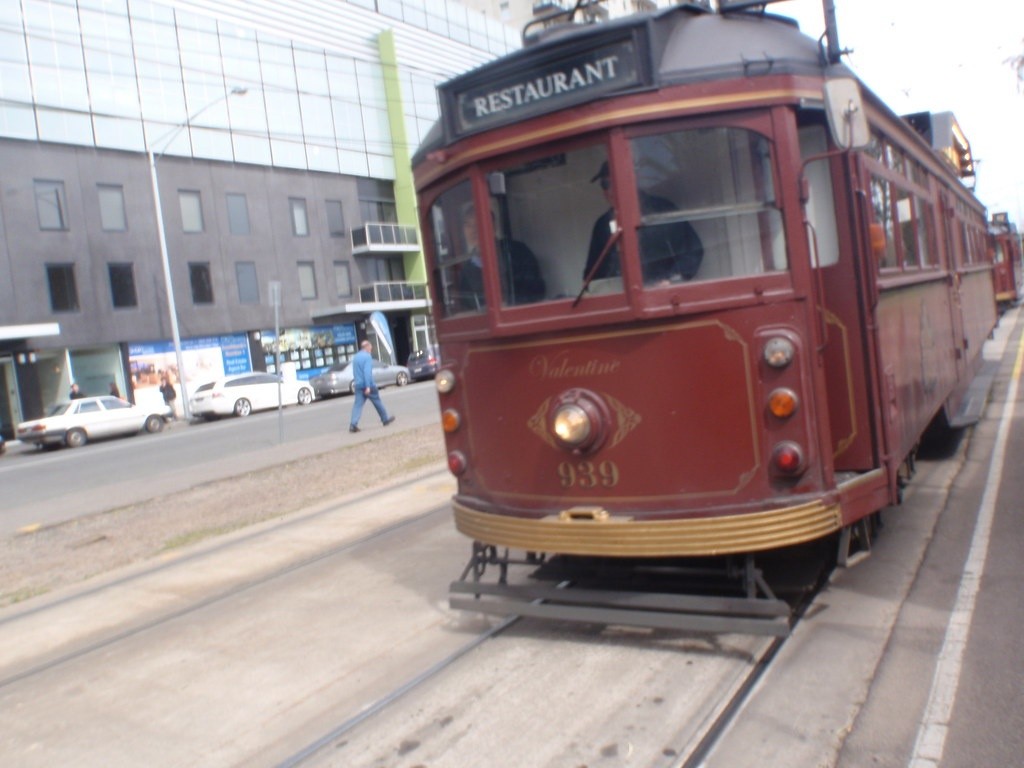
[449,200,548,316]
[69,383,84,400]
[159,377,180,423]
[581,158,705,290]
[108,382,121,400]
[348,339,397,433]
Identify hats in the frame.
[591,160,610,183]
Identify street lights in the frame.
[148,85,248,421]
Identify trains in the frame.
[408,0,1002,640]
[985,213,1023,306]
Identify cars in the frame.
[19,396,173,448]
[189,372,316,420]
[406,344,441,381]
[310,359,412,399]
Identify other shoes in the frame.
[383,417,395,425]
[350,426,361,432]
